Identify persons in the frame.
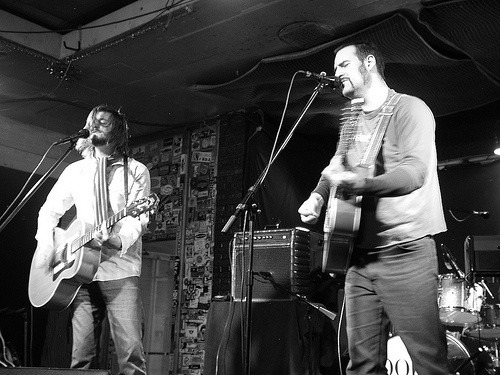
[297,41,447,375]
[35,106,151,375]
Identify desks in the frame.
[201,299,350,375]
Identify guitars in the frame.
[319,96,366,274]
[27,191,161,314]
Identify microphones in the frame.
[469,208,490,218]
[54,129,90,146]
[250,271,272,277]
[302,71,341,88]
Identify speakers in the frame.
[230,228,309,301]
[107,256,176,375]
[473,235,500,307]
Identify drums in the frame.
[459,335,500,375]
[462,304,500,343]
[438,272,486,326]
[384,329,478,375]
